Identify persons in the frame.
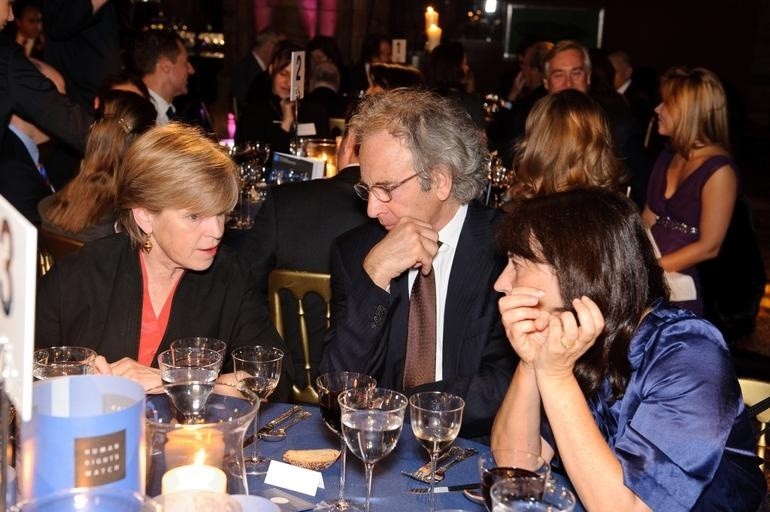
[0,0,770,512]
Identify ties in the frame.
[35,160,50,187]
[166,107,174,120]
[21,38,26,47]
[403,241,442,390]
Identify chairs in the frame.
[266,264,333,409]
[702,188,767,345]
[33,226,86,288]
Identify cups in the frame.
[6,462,17,507]
[140,378,261,497]
[14,374,147,499]
[489,476,576,512]
[480,447,552,511]
[158,335,226,425]
[7,488,163,511]
[32,345,99,380]
[229,141,308,230]
[302,137,339,178]
[231,493,281,511]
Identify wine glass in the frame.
[230,344,284,475]
[315,370,377,511]
[410,391,465,511]
[336,385,408,511]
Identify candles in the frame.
[423,6,442,29]
[425,26,446,53]
[156,458,228,498]
[161,422,226,471]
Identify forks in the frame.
[403,446,458,480]
[423,446,478,484]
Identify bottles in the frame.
[492,157,506,184]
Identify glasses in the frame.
[353,166,431,203]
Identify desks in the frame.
[0,374,603,512]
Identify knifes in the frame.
[409,483,483,495]
[243,404,302,448]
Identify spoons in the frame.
[261,411,313,440]
